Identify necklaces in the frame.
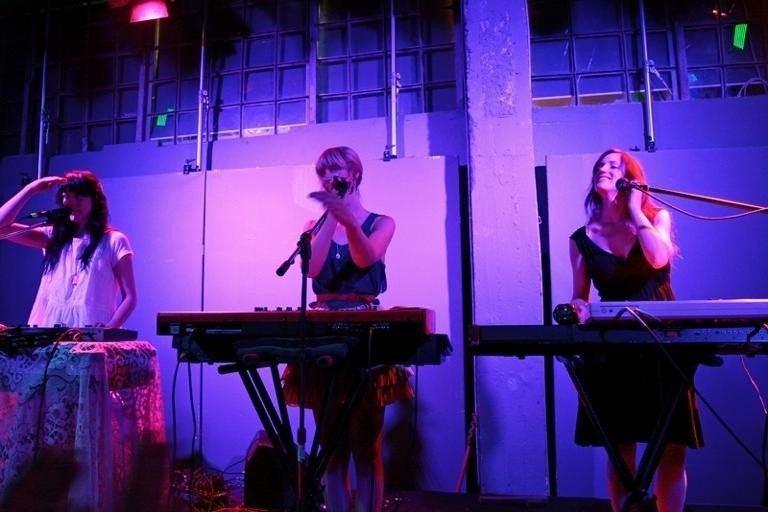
[333,220,349,260]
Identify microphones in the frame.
[615,178,649,192]
[26,207,72,220]
[331,176,354,198]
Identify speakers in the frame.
[243,429,299,512]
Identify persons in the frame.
[1,168,139,329]
[566,147,709,511]
[277,144,399,510]
[0,428,204,507]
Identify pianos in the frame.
[578,299,768,328]
[2,328,140,342]
[155,308,438,335]
